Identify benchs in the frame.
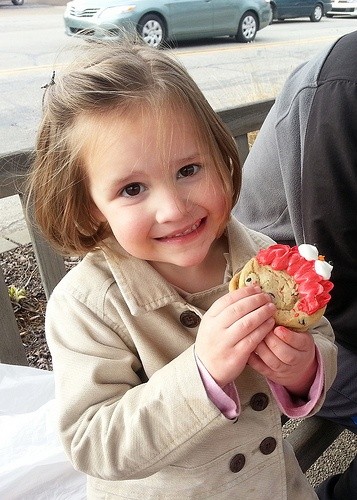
[0,98,347,474]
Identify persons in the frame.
[25,42,337,500]
[230,30,357,500]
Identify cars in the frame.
[270,0,332,23]
[63,0,273,51]
[325,0,357,18]
